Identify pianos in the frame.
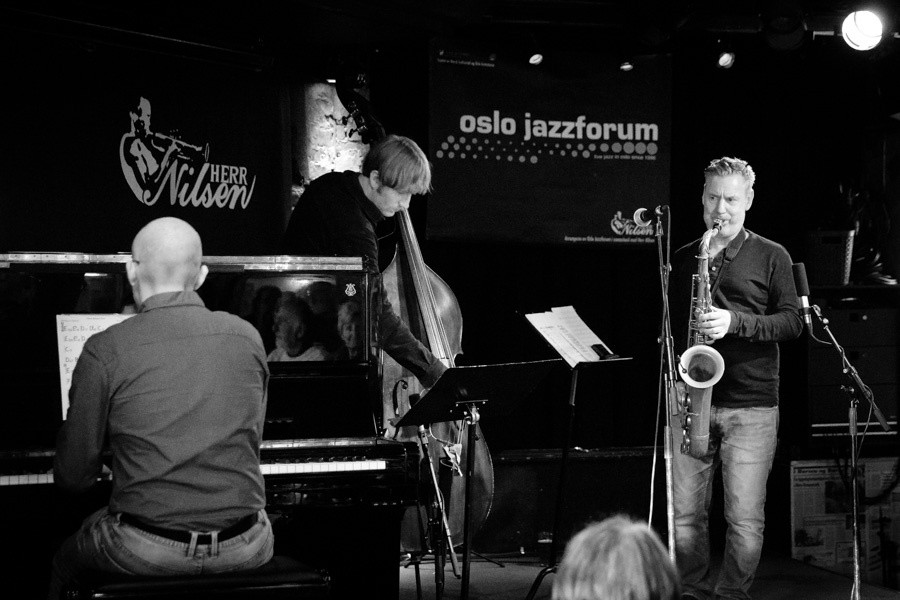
[0,246,423,600]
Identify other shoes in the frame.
[681,593,697,600]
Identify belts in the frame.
[120,513,257,545]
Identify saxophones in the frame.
[676,218,726,459]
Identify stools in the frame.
[59,561,333,600]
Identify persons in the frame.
[276,133,448,389]
[251,282,362,362]
[649,157,803,600]
[552,515,681,600]
[48,217,275,600]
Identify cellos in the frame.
[336,73,497,579]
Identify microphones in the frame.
[409,393,428,453]
[792,263,813,335]
[633,204,668,227]
